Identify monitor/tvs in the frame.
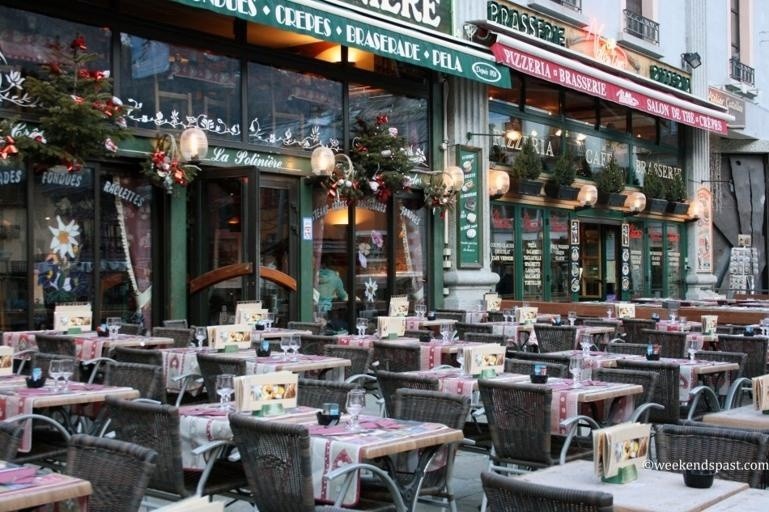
[614,303,636,319]
[700,314,718,335]
[206,300,267,350]
[751,374,769,412]
[53,304,93,332]
[232,369,300,413]
[463,342,506,375]
[377,296,409,338]
[515,306,538,323]
[0,345,13,377]
[591,421,652,480]
[484,293,502,311]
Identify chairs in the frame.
[148,42,305,144]
[0,299,769,512]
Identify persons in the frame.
[298,104,331,148]
[316,255,348,335]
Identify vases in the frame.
[0,38,136,174]
[358,230,384,303]
[146,132,200,192]
[50,215,81,258]
[330,114,457,219]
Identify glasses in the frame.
[702,179,734,192]
[681,52,702,69]
[490,170,511,200]
[576,185,599,211]
[305,147,337,186]
[437,166,464,198]
[181,129,209,166]
[624,192,647,217]
[468,129,523,141]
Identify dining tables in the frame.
[544,147,576,198]
[511,137,543,194]
[643,167,690,214]
[596,157,625,206]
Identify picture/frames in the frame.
[455,144,484,269]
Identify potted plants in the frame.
[511,137,543,194]
[643,167,690,214]
[455,144,484,269]
[544,147,576,198]
[596,157,625,206]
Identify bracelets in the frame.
[256,325,265,330]
[646,354,661,361]
[682,467,714,489]
[25,377,45,388]
[316,411,341,427]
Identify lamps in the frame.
[490,170,511,200]
[468,129,523,141]
[181,129,209,166]
[148,42,305,144]
[576,185,599,211]
[702,179,734,192]
[305,147,337,186]
[624,192,647,217]
[437,166,464,198]
[681,52,702,69]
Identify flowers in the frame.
[0,38,136,174]
[358,230,384,303]
[330,114,457,219]
[50,215,81,258]
[146,132,200,192]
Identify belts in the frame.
[195,326,207,347]
[355,304,455,342]
[503,306,614,387]
[278,334,301,361]
[214,374,234,410]
[668,308,701,361]
[345,387,365,429]
[107,316,122,338]
[262,313,275,329]
[47,358,74,393]
